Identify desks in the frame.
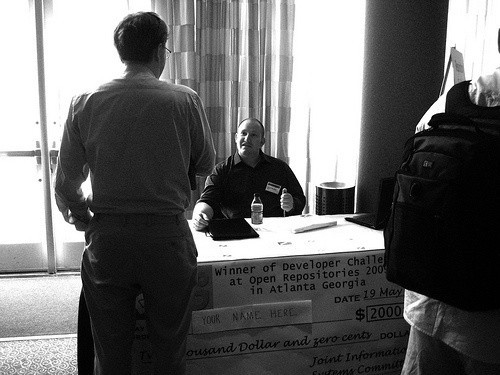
[188,212,410,375]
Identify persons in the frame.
[401,28,500,375]
[191,118,306,230]
[55,12,217,375]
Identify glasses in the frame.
[162,45,170,58]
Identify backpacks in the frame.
[380,83,500,313]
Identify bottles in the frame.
[251,193,263,232]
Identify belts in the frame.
[95,214,185,225]
